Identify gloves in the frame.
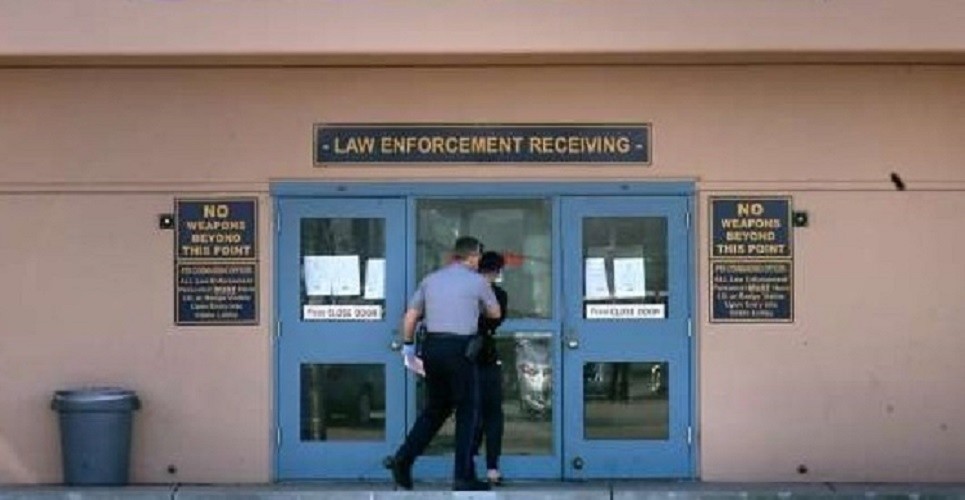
[403,342,416,356]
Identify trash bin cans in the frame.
[52,388,142,485]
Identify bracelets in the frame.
[403,342,414,345]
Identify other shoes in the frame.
[384,453,413,490]
[487,472,503,486]
[453,477,490,491]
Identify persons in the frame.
[472,250,510,488]
[607,362,630,402]
[383,235,501,491]
